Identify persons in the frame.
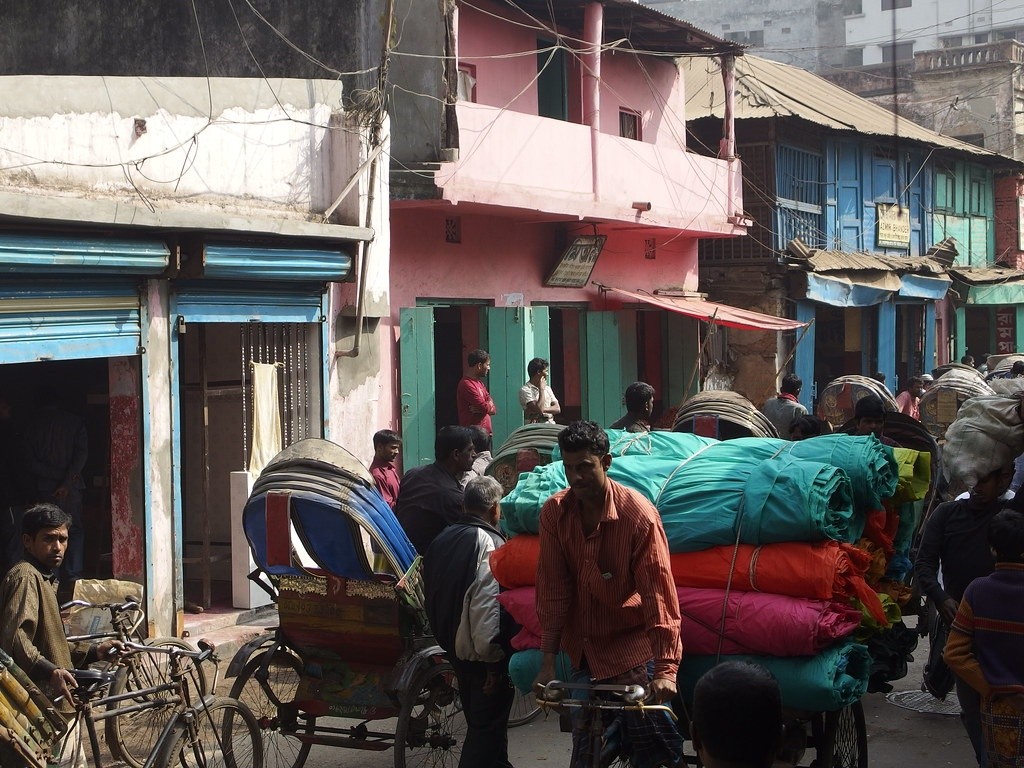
[519,357,560,424]
[395,426,478,556]
[896,374,935,421]
[531,420,685,768]
[1010,360,1024,379]
[607,382,656,432]
[874,372,886,384]
[961,355,975,367]
[368,429,401,573]
[456,349,496,437]
[459,425,494,487]
[941,507,1024,768]
[977,353,993,374]
[0,503,129,768]
[789,415,821,441]
[854,395,903,448]
[424,476,517,768]
[916,390,1024,766]
[762,374,810,440]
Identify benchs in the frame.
[275,569,435,670]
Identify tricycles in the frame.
[0,595,265,768]
[530,675,871,768]
[219,437,548,767]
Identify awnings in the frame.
[600,282,816,429]
